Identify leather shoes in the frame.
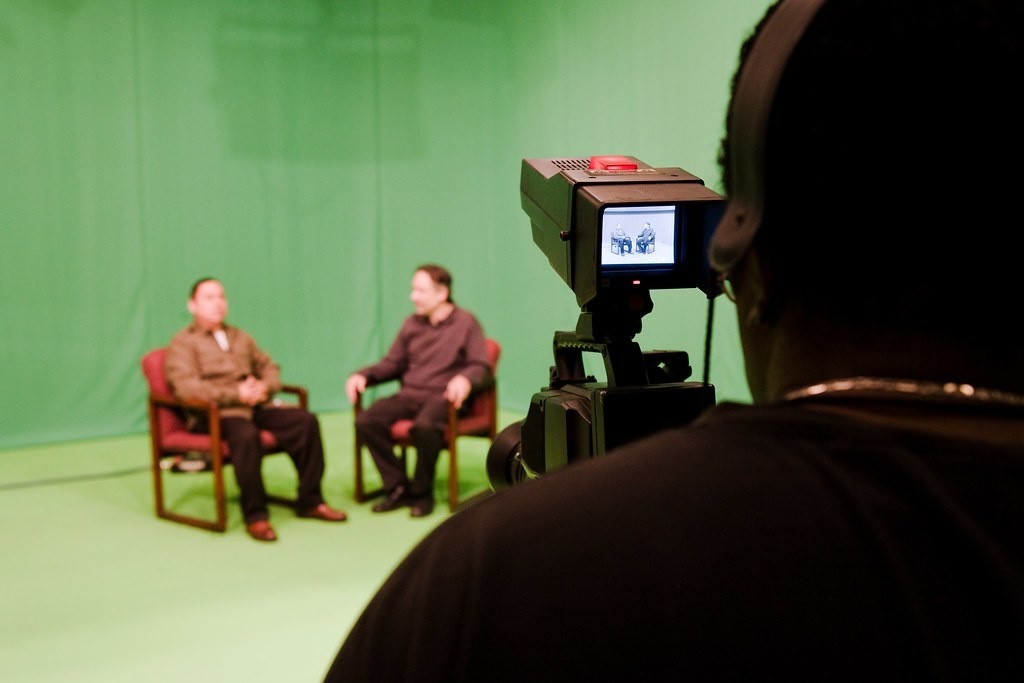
[372,481,411,512]
[247,520,276,540]
[302,503,346,521]
[411,499,434,516]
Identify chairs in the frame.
[353,339,502,514]
[141,347,309,531]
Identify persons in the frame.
[614,223,634,256]
[164,277,347,542]
[345,264,495,517]
[635,222,654,254]
[322,0,1024,683]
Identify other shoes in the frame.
[621,253,625,256]
[639,251,646,254]
[628,251,634,254]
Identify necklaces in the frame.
[781,378,1024,407]
